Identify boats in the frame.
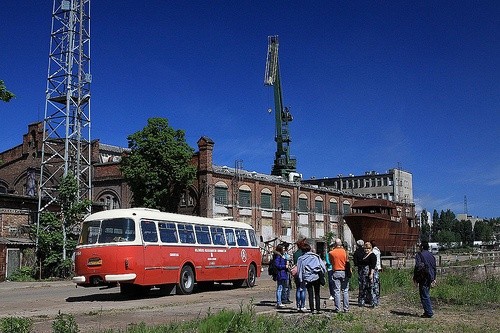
[343,197,421,251]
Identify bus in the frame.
[71,208,261,296]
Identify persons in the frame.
[293,239,308,313]
[274,244,289,308]
[360,241,377,308]
[353,240,366,273]
[413,241,437,318]
[370,240,381,296]
[281,241,293,304]
[329,238,350,314]
[324,243,337,301]
[298,244,327,314]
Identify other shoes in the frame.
[317,310,323,314]
[297,307,301,313]
[312,310,316,314]
[335,307,342,312]
[277,304,286,309]
[421,314,435,318]
[301,307,307,313]
[283,301,291,304]
[343,307,350,311]
[287,300,293,303]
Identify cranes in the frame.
[264,35,300,182]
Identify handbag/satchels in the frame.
[268,259,278,281]
[414,252,429,283]
[345,262,352,278]
[291,265,298,277]
[317,271,326,286]
